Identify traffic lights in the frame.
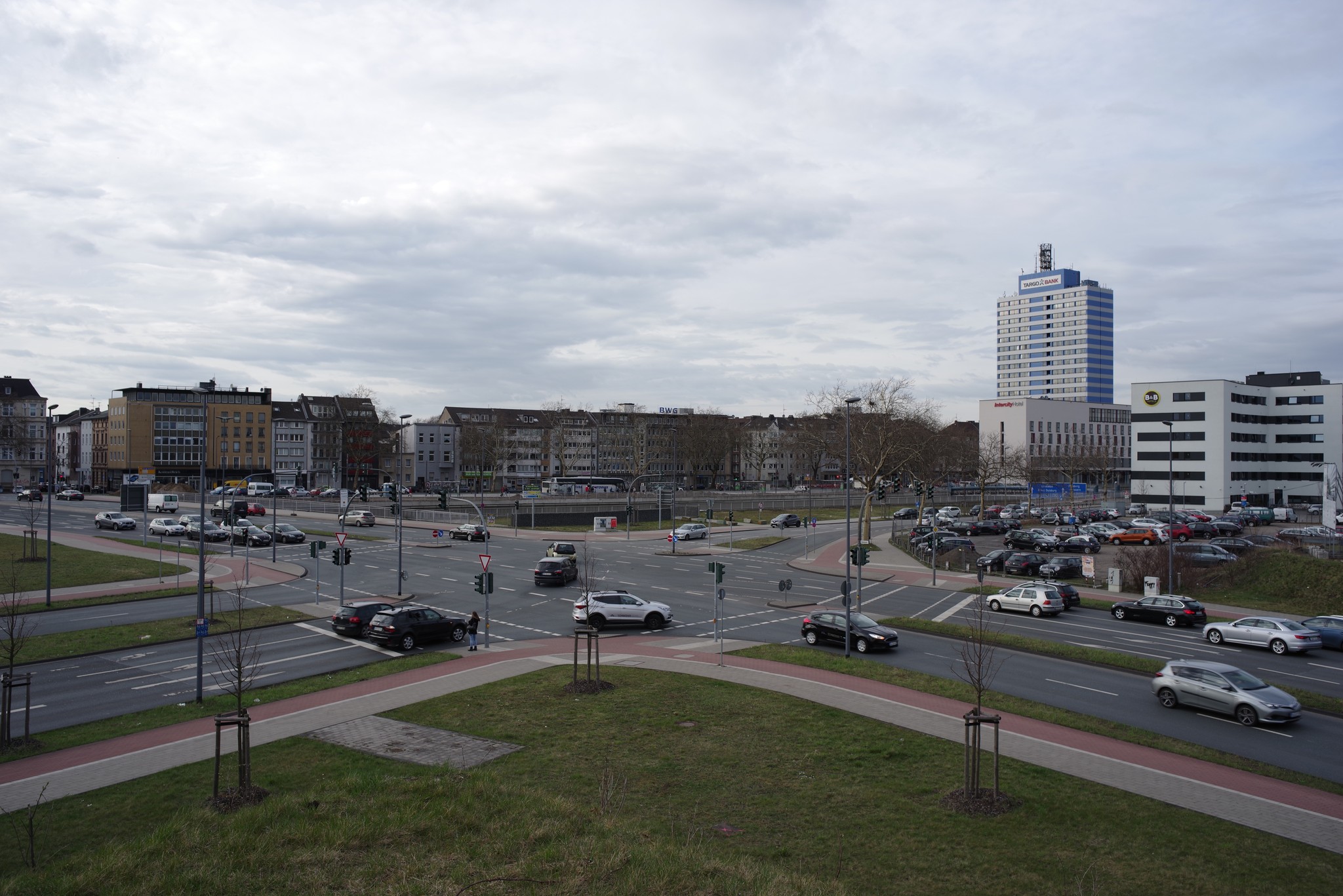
[309,541,327,558]
[388,484,397,515]
[515,500,519,510]
[334,462,338,474]
[363,463,367,475]
[296,466,301,478]
[729,511,734,521]
[242,528,248,543]
[716,563,726,584]
[875,478,934,501]
[626,505,634,515]
[438,490,447,508]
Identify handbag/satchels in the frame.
[466,618,476,630]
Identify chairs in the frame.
[1156,600,1162,604]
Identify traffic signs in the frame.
[138,466,156,480]
[1027,482,1086,502]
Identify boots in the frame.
[468,647,473,651]
[474,646,477,651]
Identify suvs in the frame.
[331,511,674,652]
[800,502,1343,728]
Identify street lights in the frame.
[1004,447,1010,507]
[1162,420,1174,596]
[215,416,234,521]
[478,427,486,526]
[189,387,209,702]
[47,404,61,606]
[397,414,413,595]
[843,395,864,661]
[272,418,286,563]
[668,427,676,536]
[1183,481,1187,510]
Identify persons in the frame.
[379,487,383,497]
[502,486,509,497]
[616,485,636,492]
[604,487,606,492]
[468,611,481,651]
[408,487,412,497]
[610,487,612,492]
[585,486,596,494]
[567,486,571,496]
[639,485,647,494]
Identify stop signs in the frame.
[667,535,673,542]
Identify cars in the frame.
[670,523,709,541]
[9,469,1021,508]
[770,513,801,528]
[55,490,84,501]
[94,500,306,548]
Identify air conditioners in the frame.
[617,470,621,474]
[682,471,685,473]
[626,470,630,473]
[621,470,625,474]
[613,471,617,473]
[608,471,612,473]
[690,470,693,474]
[677,471,681,473]
[656,470,660,473]
[648,470,652,474]
